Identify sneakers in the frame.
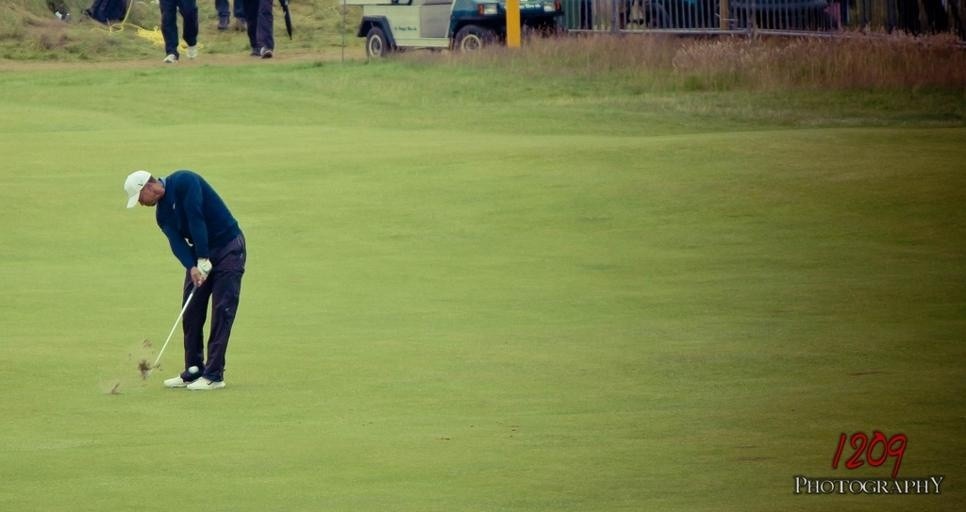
[187,378,226,389]
[186,45,196,59]
[252,48,260,55]
[164,377,186,388]
[217,15,230,28]
[260,46,272,57]
[165,54,177,62]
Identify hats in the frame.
[124,170,151,208]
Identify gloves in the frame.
[198,257,213,280]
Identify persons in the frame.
[244,1,289,59]
[214,1,247,32]
[124,169,247,391]
[157,0,201,65]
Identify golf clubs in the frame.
[140,285,196,371]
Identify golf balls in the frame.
[188,366,198,374]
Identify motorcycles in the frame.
[355,0,563,59]
